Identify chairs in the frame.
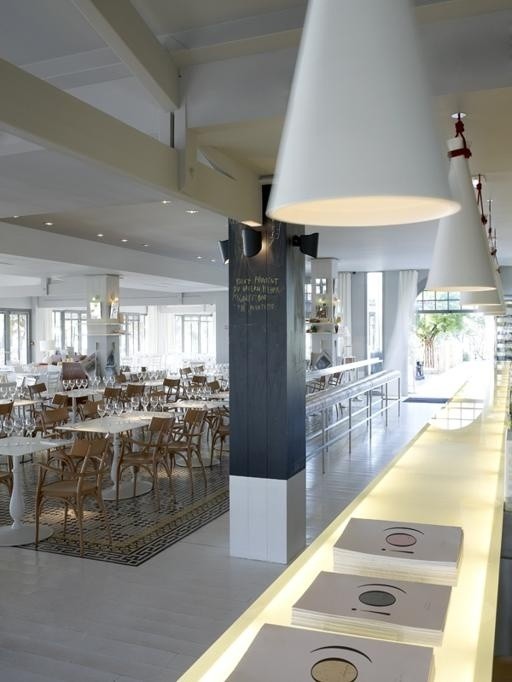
[0,360,228,557]
[307,354,357,396]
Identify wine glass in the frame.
[110,376,115,388]
[159,395,167,413]
[147,372,153,383]
[186,387,193,402]
[95,376,100,390]
[0,386,8,401]
[221,380,228,392]
[150,396,158,415]
[63,380,69,392]
[199,387,206,401]
[82,379,89,389]
[153,372,158,380]
[16,387,22,402]
[106,403,114,426]
[89,377,95,390]
[140,397,149,415]
[142,372,147,383]
[137,372,142,382]
[130,397,139,415]
[103,375,110,387]
[69,380,76,392]
[96,404,106,426]
[193,386,200,402]
[0,418,3,446]
[9,386,17,401]
[124,402,132,422]
[3,418,14,446]
[205,387,211,402]
[25,418,36,446]
[76,379,82,389]
[14,418,24,446]
[113,403,123,424]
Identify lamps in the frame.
[266,0,462,227]
[422,115,497,293]
[291,232,319,260]
[218,240,229,265]
[458,198,507,316]
[240,227,263,258]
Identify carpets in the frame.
[0,445,230,567]
